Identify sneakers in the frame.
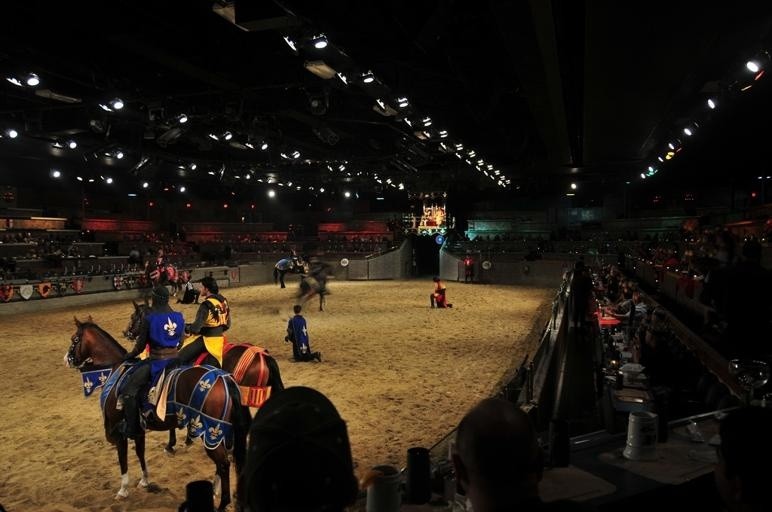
[316,351,323,362]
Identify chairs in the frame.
[446,242,770,511]
[3,241,400,305]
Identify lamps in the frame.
[284,24,511,190]
[2,70,450,203]
[640,48,770,181]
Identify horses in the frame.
[273,257,311,288]
[63,312,252,512]
[289,264,337,311]
[145,253,183,298]
[122,293,284,462]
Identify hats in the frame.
[152,286,170,298]
[202,277,219,292]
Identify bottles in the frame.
[623,410,660,462]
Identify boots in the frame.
[115,400,141,437]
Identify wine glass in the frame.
[728,359,770,406]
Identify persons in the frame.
[0,216,771,510]
